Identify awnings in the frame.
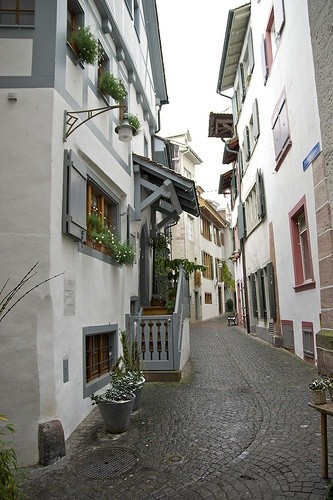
[216,3,251,102]
[207,112,239,196]
[133,154,200,227]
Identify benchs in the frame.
[308,400,333,479]
[227,312,240,326]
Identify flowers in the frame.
[85,207,138,264]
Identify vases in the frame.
[85,238,115,257]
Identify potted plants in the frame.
[325,375,333,403]
[91,363,136,434]
[108,329,146,412]
[309,379,327,405]
[72,27,97,66]
[98,69,128,101]
[129,114,140,135]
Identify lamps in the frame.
[63,105,139,143]
